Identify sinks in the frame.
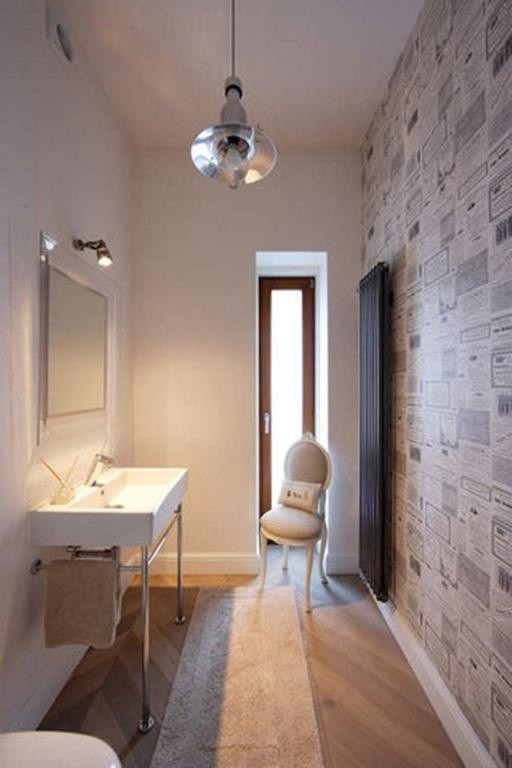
[65,466,186,513]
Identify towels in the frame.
[45,559,121,650]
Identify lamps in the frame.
[191,2,278,190]
[72,235,113,267]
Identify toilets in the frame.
[0,731,122,768]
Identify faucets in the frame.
[83,452,116,486]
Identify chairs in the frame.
[261,432,332,613]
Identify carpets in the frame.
[148,585,326,768]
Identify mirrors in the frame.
[38,232,108,423]
